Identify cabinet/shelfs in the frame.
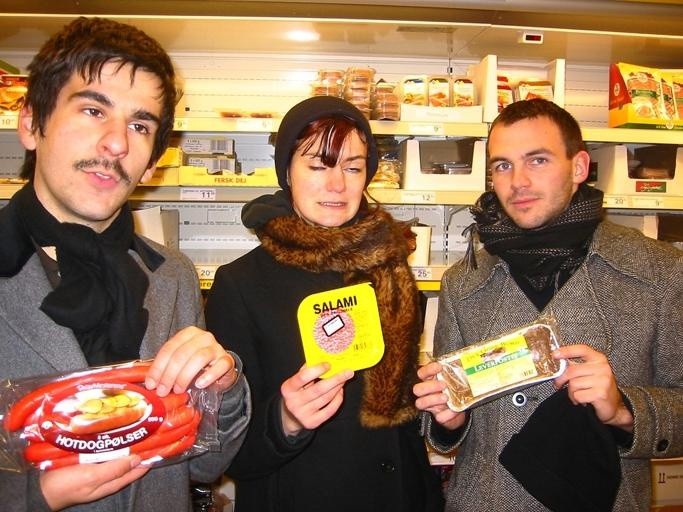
[0,54,682,291]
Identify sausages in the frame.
[2,363,201,470]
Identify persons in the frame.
[0,17,252,512]
[203,96,443,510]
[412,97,683,511]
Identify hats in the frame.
[274,95,379,198]
[496,388,622,512]
